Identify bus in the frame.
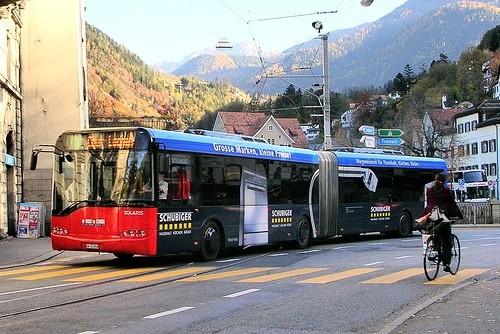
[30,126,447,262]
[447,169,490,203]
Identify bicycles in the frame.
[423,215,461,281]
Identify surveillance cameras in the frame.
[312,20,323,33]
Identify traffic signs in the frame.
[378,138,405,146]
[378,128,404,137]
[360,137,377,147]
[359,125,376,136]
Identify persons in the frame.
[142,173,168,200]
[418,173,462,272]
